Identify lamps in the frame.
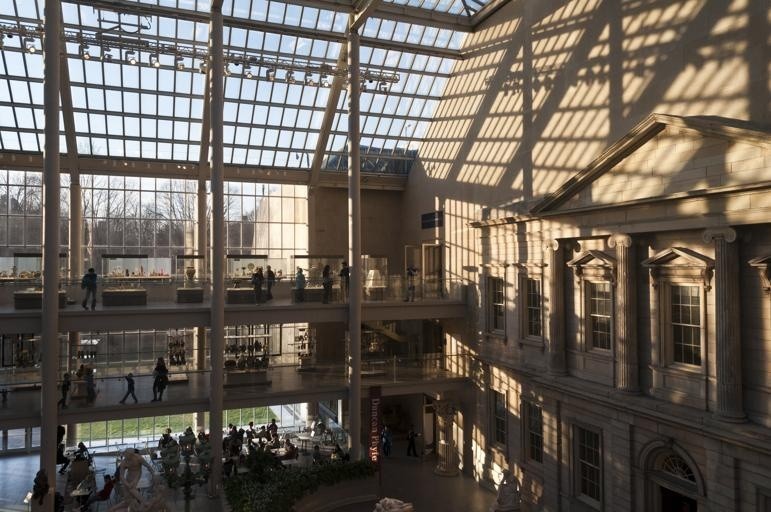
[1,22,402,98]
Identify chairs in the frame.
[49,426,339,512]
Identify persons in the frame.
[380,424,422,458]
[250,261,349,306]
[159,428,173,450]
[76,364,84,378]
[151,356,168,402]
[79,474,114,512]
[119,448,158,511]
[119,373,137,404]
[57,373,71,408]
[402,264,418,302]
[56,444,70,475]
[72,442,89,462]
[80,268,97,311]
[222,418,345,478]
